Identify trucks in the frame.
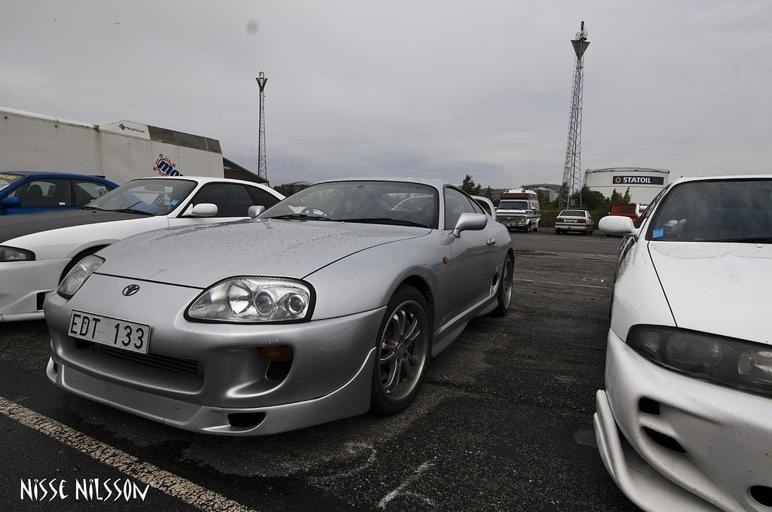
[611,204,649,227]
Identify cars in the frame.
[555,209,594,236]
[392,195,496,224]
[43,177,516,435]
[1,177,327,321]
[0,168,161,219]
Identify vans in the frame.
[593,174,767,510]
[495,187,542,233]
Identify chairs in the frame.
[19,185,42,209]
